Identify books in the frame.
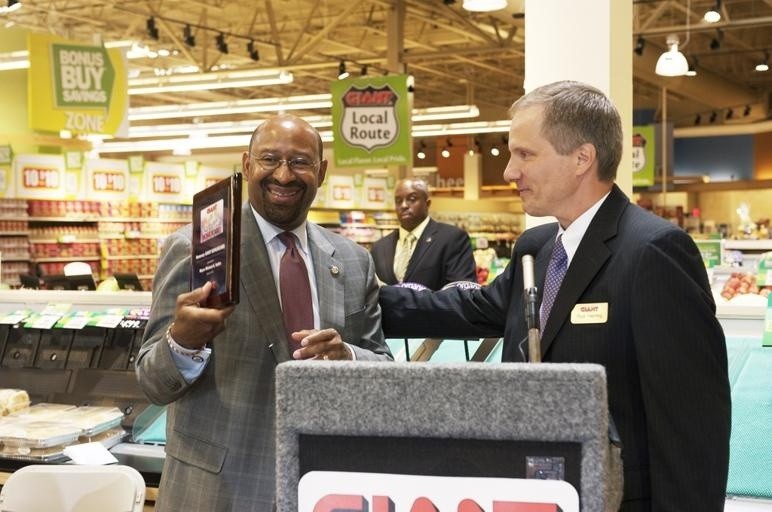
[189,171,241,306]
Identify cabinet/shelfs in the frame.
[309,200,525,266]
[1,200,193,294]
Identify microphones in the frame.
[522,254,540,330]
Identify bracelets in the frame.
[164,323,207,357]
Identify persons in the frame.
[370,178,476,290]
[375,78,731,511]
[136,114,392,512]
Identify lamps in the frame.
[214,32,227,55]
[246,40,261,60]
[180,24,196,47]
[653,36,691,76]
[360,65,368,77]
[129,63,416,122]
[59,82,514,154]
[145,15,158,40]
[338,60,349,78]
[1,38,184,72]
[635,28,727,57]
[128,75,296,90]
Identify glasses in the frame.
[251,153,319,176]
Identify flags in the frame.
[632,125,655,189]
[329,75,412,169]
[27,31,132,139]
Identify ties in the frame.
[541,234,568,335]
[275,233,313,350]
[396,235,416,283]
